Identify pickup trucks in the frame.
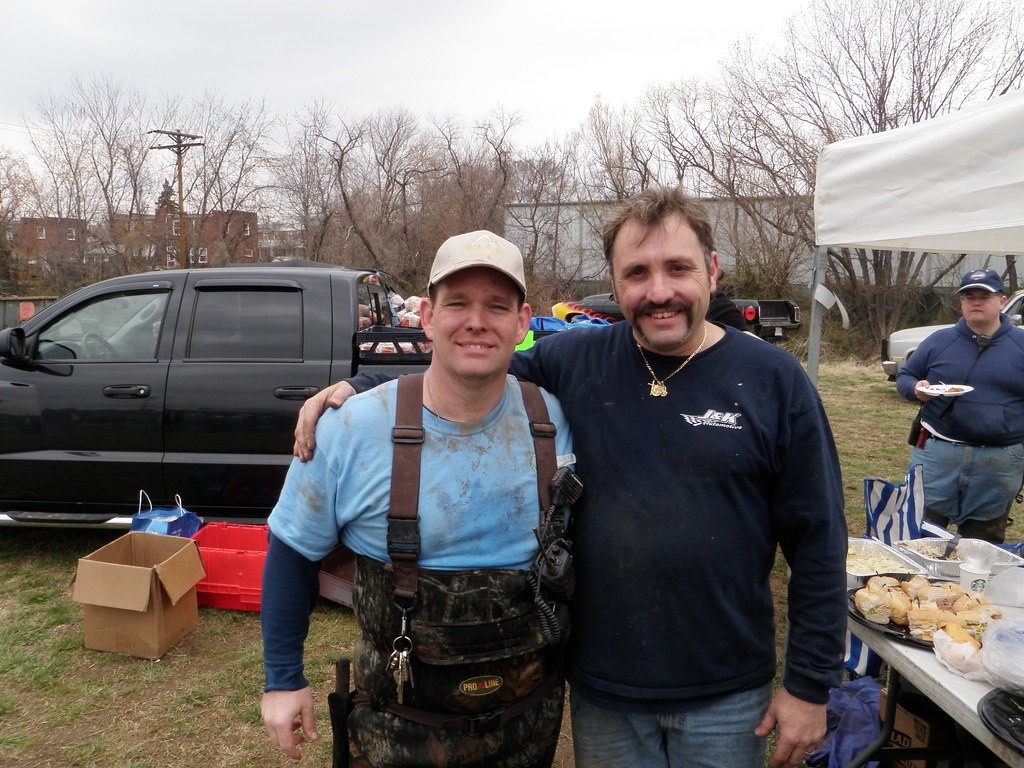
[1,262,563,531]
[550,291,803,342]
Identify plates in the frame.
[916,384,974,397]
[846,584,944,650]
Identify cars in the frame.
[880,289,1024,383]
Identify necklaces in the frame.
[427,369,439,417]
[638,324,707,397]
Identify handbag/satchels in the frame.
[130,489,203,538]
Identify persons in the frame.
[705,268,751,332]
[294,186,849,768]
[897,270,1024,543]
[261,230,576,768]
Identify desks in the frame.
[786,568,1024,768]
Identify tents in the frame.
[809,89,1024,388]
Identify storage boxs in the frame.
[188,522,268,612]
[68,531,207,661]
[880,687,959,768]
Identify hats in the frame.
[955,268,1004,295]
[426,229,528,305]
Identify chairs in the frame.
[844,463,1024,682]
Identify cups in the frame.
[959,562,991,597]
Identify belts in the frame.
[927,431,975,447]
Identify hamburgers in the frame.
[854,575,1005,650]
